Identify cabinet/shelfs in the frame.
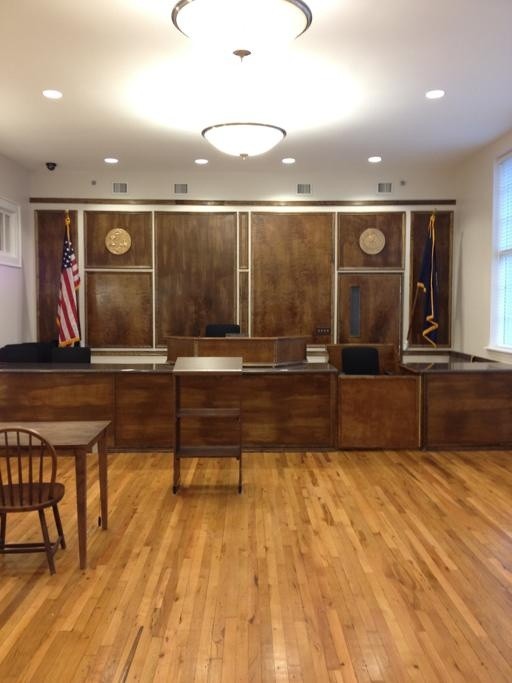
[173,356,243,493]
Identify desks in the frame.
[0,421,110,570]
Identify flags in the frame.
[56,226,81,349]
[416,214,441,349]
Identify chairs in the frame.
[0,427,66,575]
[205,324,240,337]
[341,346,379,375]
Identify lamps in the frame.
[171,0,312,63]
[201,122,287,160]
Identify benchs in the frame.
[0,347,91,369]
